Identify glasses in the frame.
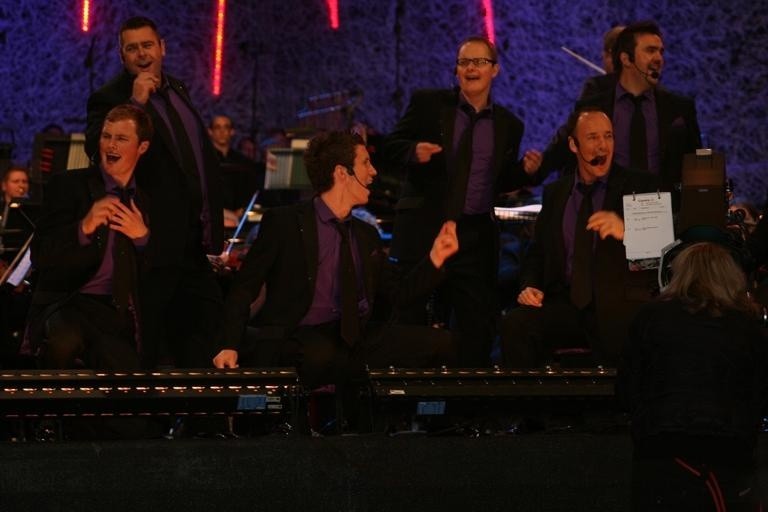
[455,58,496,67]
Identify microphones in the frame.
[452,75,462,93]
[634,64,659,78]
[351,171,376,190]
[574,141,602,165]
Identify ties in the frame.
[158,87,204,215]
[571,183,601,310]
[114,191,133,322]
[445,107,490,218]
[627,95,648,171]
[335,223,361,348]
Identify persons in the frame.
[504,22,704,368]
[624,242,768,511]
[209,127,461,369]
[388,37,543,368]
[1,14,266,372]
